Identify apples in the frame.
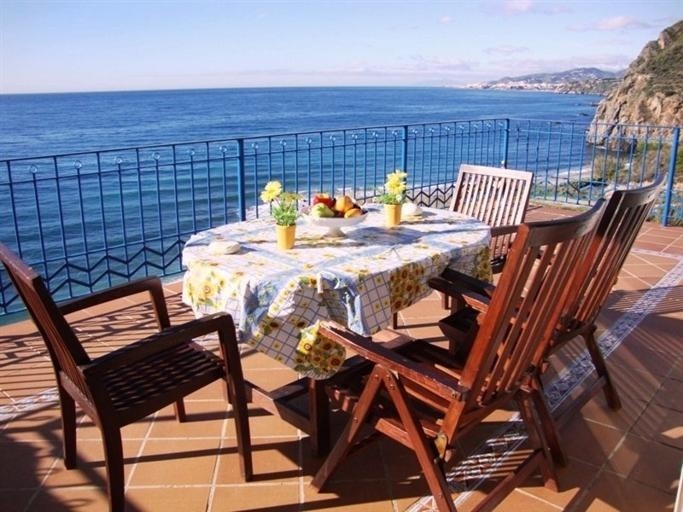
[313,193,333,208]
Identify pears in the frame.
[313,203,334,217]
[333,196,353,212]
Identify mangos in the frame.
[344,208,362,218]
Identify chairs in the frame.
[437,170,668,469]
[1,242,256,512]
[300,196,607,511]
[438,162,536,313]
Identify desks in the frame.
[174,200,494,450]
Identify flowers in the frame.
[374,167,413,207]
[261,177,304,225]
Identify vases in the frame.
[380,201,408,228]
[273,219,299,252]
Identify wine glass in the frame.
[301,208,368,238]
